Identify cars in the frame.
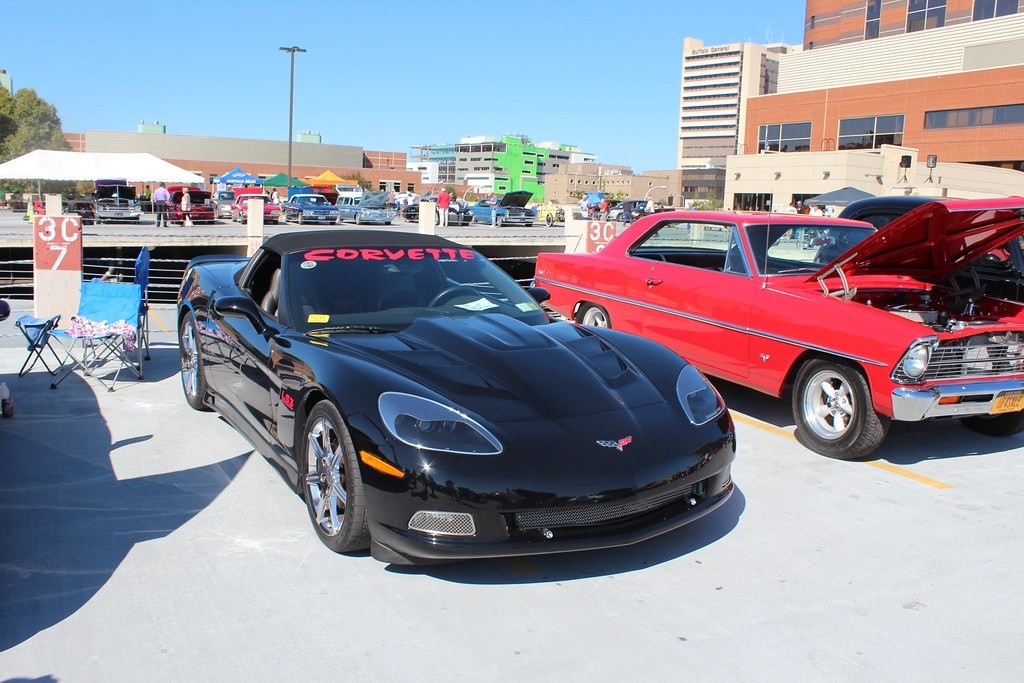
[403,195,474,227]
[63,199,96,226]
[533,199,565,227]
[93,180,142,226]
[281,187,341,225]
[335,189,399,226]
[215,190,236,220]
[467,190,536,228]
[812,197,1023,303]
[165,184,216,225]
[231,186,281,225]
[543,194,1024,463]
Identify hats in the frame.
[488,191,493,196]
[442,188,446,191]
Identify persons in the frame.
[450,196,468,225]
[386,191,397,208]
[407,193,415,205]
[808,205,823,246]
[486,192,499,228]
[181,188,190,227]
[623,200,634,226]
[228,185,234,197]
[263,188,280,204]
[436,187,453,227]
[145,186,152,212]
[154,183,170,227]
[687,203,699,233]
[646,195,659,237]
[577,196,619,221]
[532,204,540,218]
[785,201,804,240]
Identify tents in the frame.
[0,149,206,205]
[212,168,359,199]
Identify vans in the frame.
[336,185,364,199]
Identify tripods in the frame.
[899,168,908,183]
[924,168,934,184]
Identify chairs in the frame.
[373,257,445,310]
[260,259,320,320]
[46,247,150,391]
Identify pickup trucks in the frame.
[606,199,676,224]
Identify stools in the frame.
[15,315,64,378]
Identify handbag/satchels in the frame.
[186,215,194,226]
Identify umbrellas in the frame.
[805,187,877,207]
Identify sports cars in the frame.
[177,226,737,568]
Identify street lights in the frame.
[278,46,308,202]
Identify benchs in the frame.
[629,253,780,269]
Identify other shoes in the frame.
[490,226,495,228]
[164,225,169,227]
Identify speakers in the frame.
[901,156,911,168]
[927,155,937,168]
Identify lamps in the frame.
[924,155,937,182]
[865,173,883,178]
[898,155,912,183]
